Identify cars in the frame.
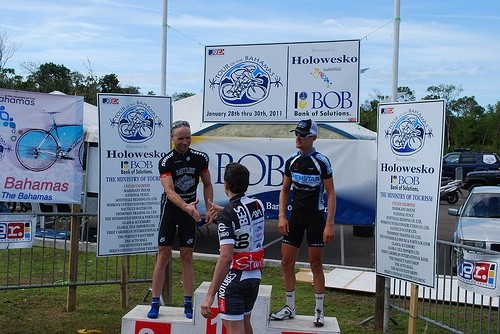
[461,171,500,189]
[448,186,500,272]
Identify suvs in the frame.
[442,150,500,176]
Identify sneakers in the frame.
[270,305,296,320]
[313,309,325,327]
[184,302,193,318]
[147,303,160,318]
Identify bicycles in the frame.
[14,112,83,172]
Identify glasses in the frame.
[294,130,314,139]
[170,121,190,133]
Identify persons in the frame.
[269,118,336,327]
[147,121,214,320]
[201,162,266,334]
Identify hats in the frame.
[290,119,318,136]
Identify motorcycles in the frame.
[440,177,464,204]
